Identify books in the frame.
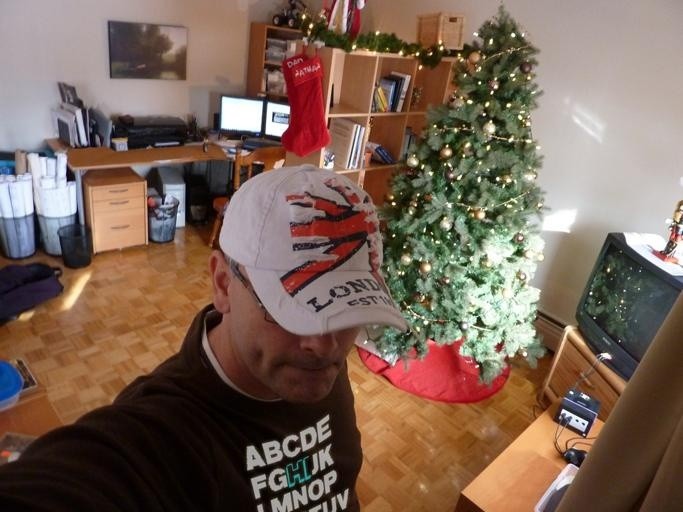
[326,117,358,170]
[346,124,362,169]
[50,80,116,151]
[351,126,365,168]
[370,142,393,163]
[370,70,412,114]
[366,144,391,164]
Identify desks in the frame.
[453,400,605,512]
[44,137,266,251]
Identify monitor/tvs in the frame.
[264,99,291,142]
[575,232,683,382]
[219,94,265,140]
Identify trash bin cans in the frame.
[148,195,179,243]
[57,225,91,269]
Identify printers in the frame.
[116,115,186,149]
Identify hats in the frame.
[220,164,411,338]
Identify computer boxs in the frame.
[156,167,187,228]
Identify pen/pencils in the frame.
[191,113,196,124]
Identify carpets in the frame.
[356,340,512,402]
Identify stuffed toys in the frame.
[272,0,307,29]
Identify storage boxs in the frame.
[0,360,24,411]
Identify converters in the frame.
[555,389,600,438]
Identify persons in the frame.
[0,163,405,512]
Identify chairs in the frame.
[208,142,285,249]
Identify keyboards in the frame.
[243,142,270,151]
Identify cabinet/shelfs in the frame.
[285,42,468,218]
[537,323,628,422]
[81,167,149,255]
[248,23,305,102]
